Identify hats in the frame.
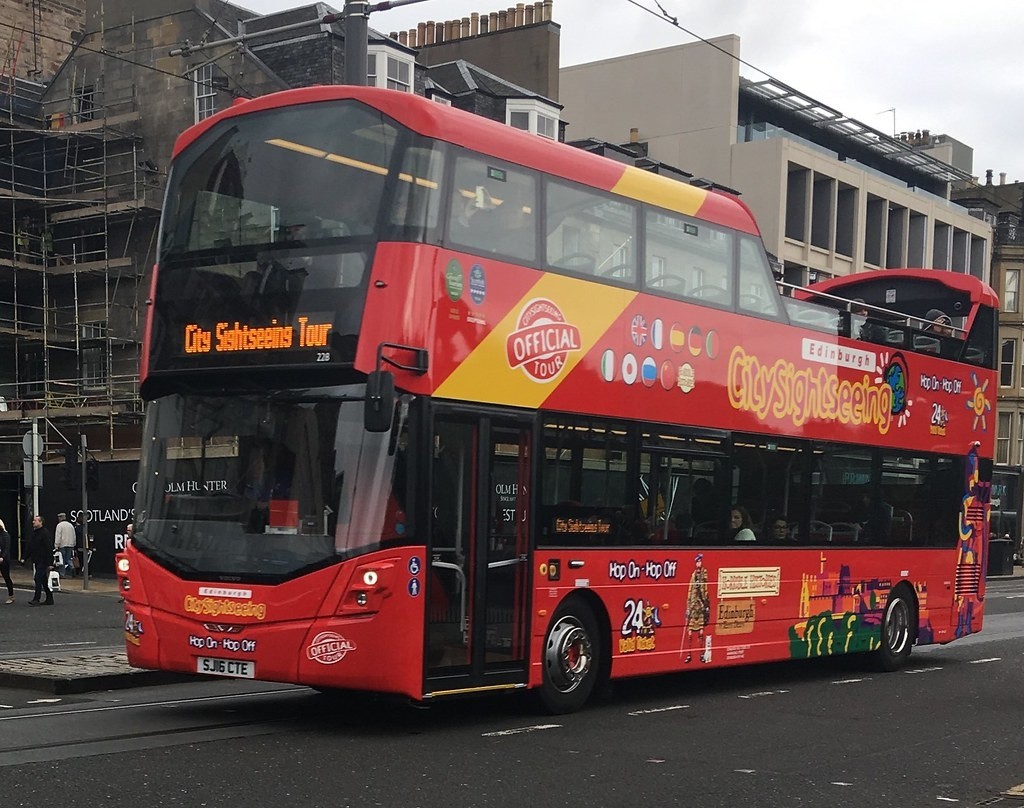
[57,513,66,517]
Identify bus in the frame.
[113,83,1009,718]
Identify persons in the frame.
[444,188,487,242]
[20,516,54,606]
[238,208,340,294]
[916,309,953,337]
[691,477,717,527]
[0,519,18,605]
[761,513,799,546]
[71,516,93,581]
[729,506,756,541]
[116,524,133,606]
[51,511,77,581]
[847,296,887,343]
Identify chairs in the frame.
[248,229,987,566]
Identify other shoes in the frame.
[6,595,15,603]
[40,600,54,605]
[27,600,41,606]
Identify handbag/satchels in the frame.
[73,551,80,568]
[47,568,61,592]
[52,549,63,566]
